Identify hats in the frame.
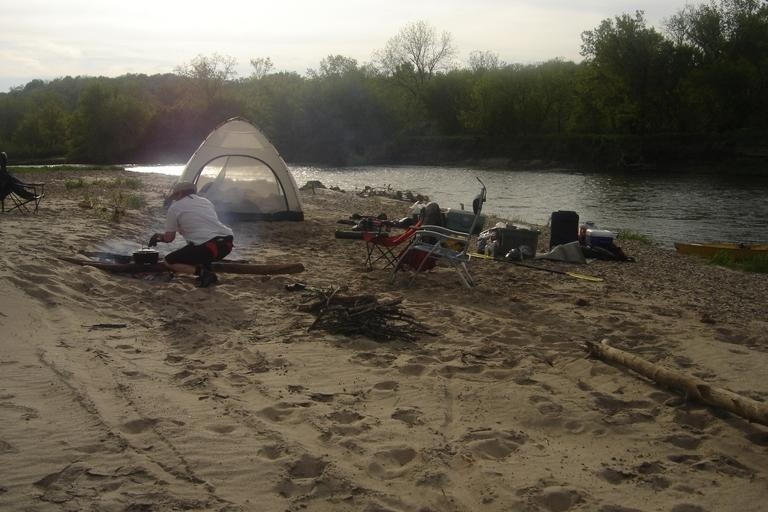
[168,182,197,200]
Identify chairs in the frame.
[0,182,45,216]
[364,177,487,288]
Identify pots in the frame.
[132,240,158,265]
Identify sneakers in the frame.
[200,273,217,288]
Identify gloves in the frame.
[148,233,165,247]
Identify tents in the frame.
[169,115,304,222]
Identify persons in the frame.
[149,181,234,288]
[0,152,40,201]
